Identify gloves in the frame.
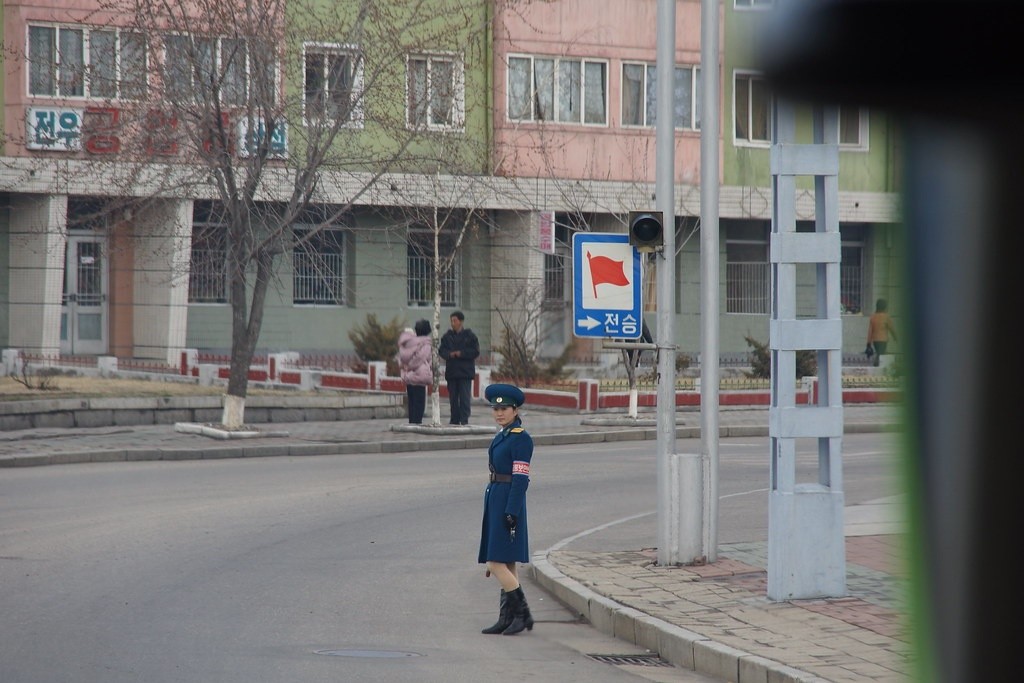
[505,514,517,527]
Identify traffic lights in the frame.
[628,211,666,249]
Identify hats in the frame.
[485,384,525,408]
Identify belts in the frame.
[489,473,513,482]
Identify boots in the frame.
[502,583,533,635]
[482,589,514,634]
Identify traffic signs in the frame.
[569,232,646,339]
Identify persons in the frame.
[438,310,480,425]
[614,318,653,367]
[479,384,534,634]
[866,299,899,367]
[399,320,432,423]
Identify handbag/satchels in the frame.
[864,344,874,358]
